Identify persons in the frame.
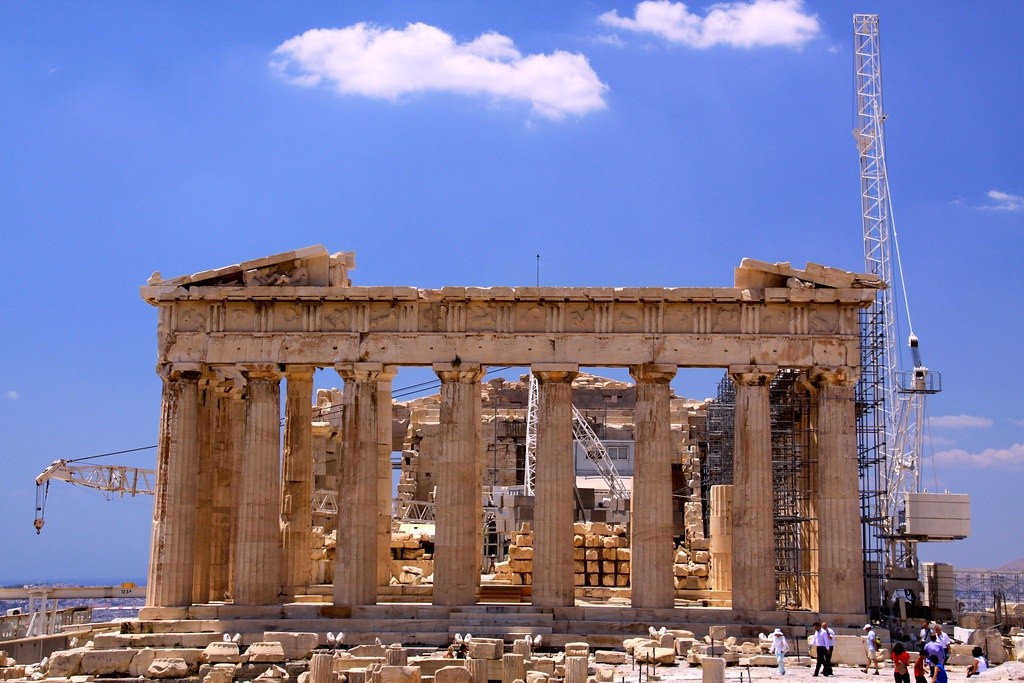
[861,624,881,675]
[967,646,987,678]
[891,643,912,683]
[913,621,951,683]
[456,644,467,659]
[808,622,835,677]
[443,646,454,658]
[768,628,790,675]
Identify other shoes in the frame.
[828,673,833,675]
[782,671,786,675]
[861,670,867,674]
[813,674,818,676]
[821,672,824,674]
[873,671,879,675]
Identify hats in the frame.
[972,647,984,657]
[863,623,874,630]
[773,628,783,635]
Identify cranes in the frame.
[34,359,631,535]
[853,14,971,627]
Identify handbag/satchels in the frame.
[875,634,882,649]
[780,636,790,653]
